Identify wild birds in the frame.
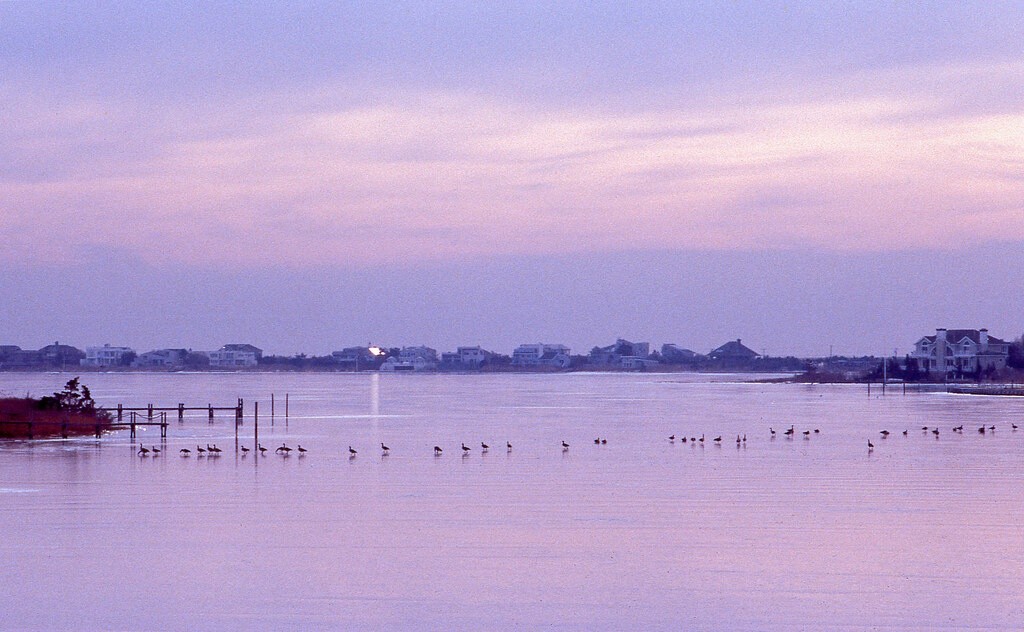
[349,446,357,454]
[462,443,471,451]
[562,441,570,450]
[276,443,307,455]
[668,434,746,443]
[879,424,1017,434]
[206,443,221,454]
[768,425,820,437]
[434,446,442,452]
[180,449,190,455]
[258,444,267,453]
[506,441,511,448]
[481,442,489,450]
[381,443,390,451]
[593,438,607,443]
[138,443,149,455]
[240,446,249,453]
[867,439,874,448]
[152,446,162,454]
[197,446,206,454]
